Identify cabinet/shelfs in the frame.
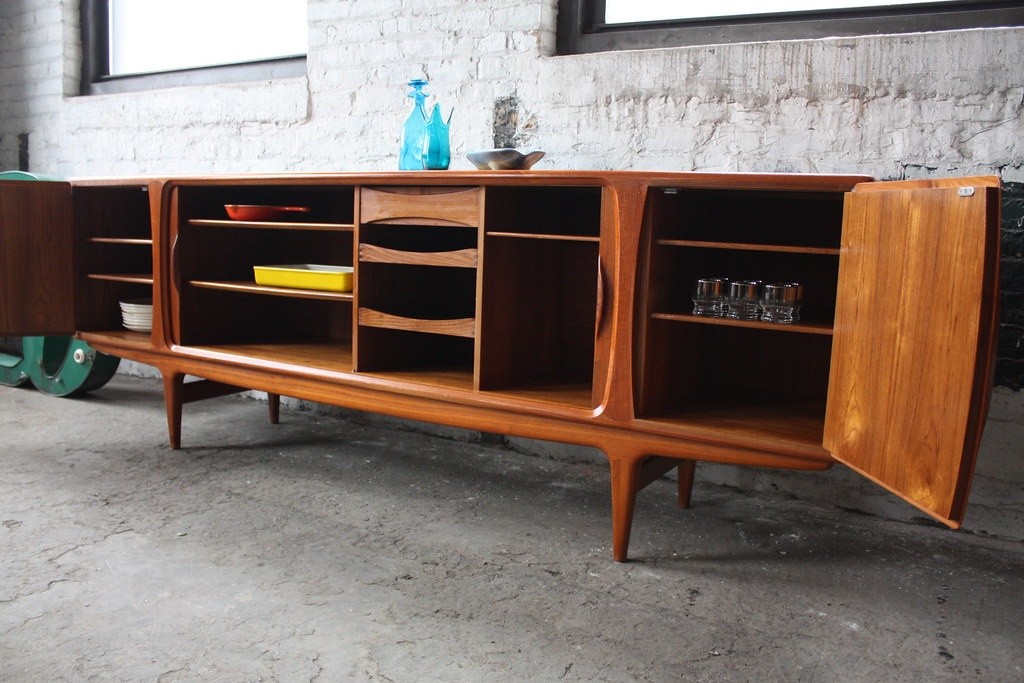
[0,176,1002,562]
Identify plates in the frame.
[118,298,152,333]
[466,148,546,170]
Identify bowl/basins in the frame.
[223,204,308,222]
